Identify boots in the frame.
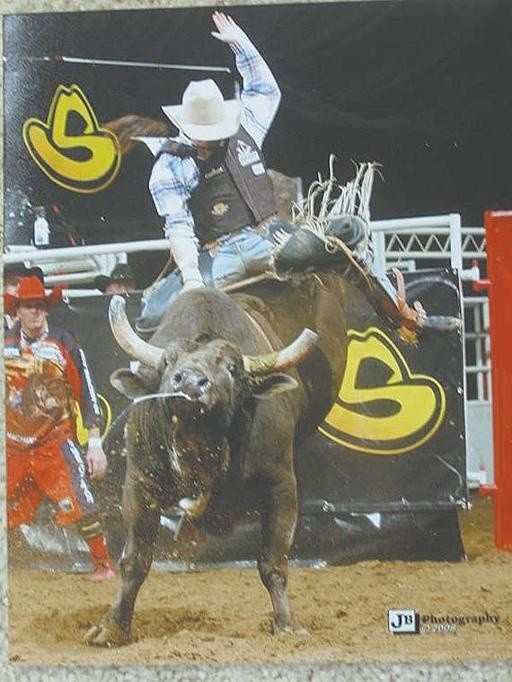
[78,518,117,584]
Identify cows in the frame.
[78,114,377,650]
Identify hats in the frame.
[159,77,242,143]
[2,275,68,318]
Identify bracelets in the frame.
[88,437,102,449]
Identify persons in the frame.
[108,9,461,402]
[5,272,117,586]
[94,261,150,294]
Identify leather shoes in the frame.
[397,314,463,346]
